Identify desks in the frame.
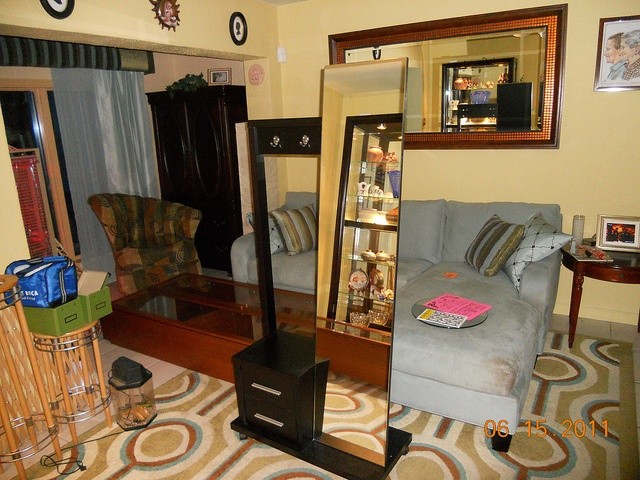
[561,233,640,349]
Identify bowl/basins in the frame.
[368,308,390,325]
[468,91,491,103]
[349,310,372,328]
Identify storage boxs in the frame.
[76,286,112,324]
[22,298,85,338]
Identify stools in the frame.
[0,275,62,480]
[31,319,112,455]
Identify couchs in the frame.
[230,190,563,434]
[87,194,204,297]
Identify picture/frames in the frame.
[207,68,232,86]
[39,0,76,20]
[229,11,248,46]
[594,214,640,252]
[592,14,640,92]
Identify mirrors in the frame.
[327,2,569,151]
[311,59,409,472]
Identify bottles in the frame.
[571,215,586,244]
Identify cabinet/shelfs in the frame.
[145,84,248,272]
[230,329,330,452]
[324,112,401,342]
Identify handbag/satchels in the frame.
[3,256,78,308]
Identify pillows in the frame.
[499,212,574,295]
[271,205,316,257]
[464,214,525,276]
[248,212,283,256]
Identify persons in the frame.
[620,30,640,80]
[605,32,630,83]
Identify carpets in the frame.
[5,329,639,479]
